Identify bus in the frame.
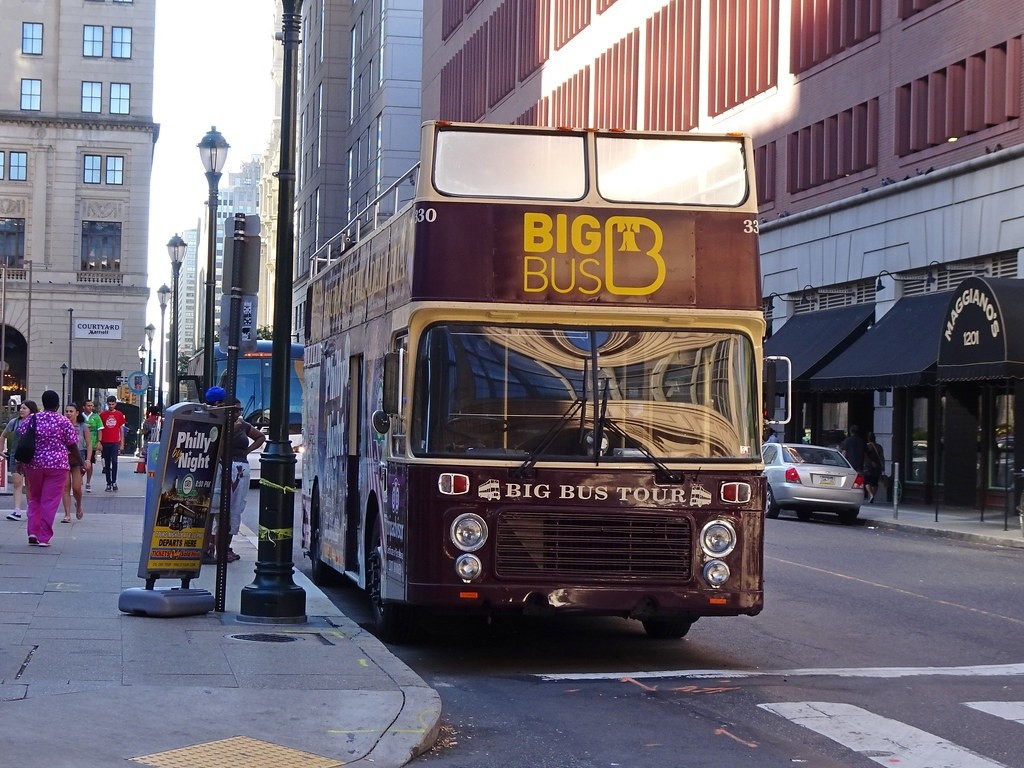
[171,338,305,491]
[295,117,795,644]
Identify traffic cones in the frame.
[133,455,146,474]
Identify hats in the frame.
[206,386,233,401]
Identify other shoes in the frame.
[226,547,240,563]
[106,485,111,491]
[28,535,39,544]
[203,548,217,564]
[102,468,112,473]
[6,512,21,521]
[86,485,91,491]
[39,542,51,547]
[112,483,118,491]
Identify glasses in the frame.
[234,407,243,413]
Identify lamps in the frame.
[927,261,989,282]
[801,284,856,304]
[766,292,818,311]
[875,270,927,291]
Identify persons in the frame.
[16,391,88,547]
[61,403,92,522]
[101,451,112,473]
[99,396,125,491]
[843,427,885,503]
[202,388,266,564]
[82,400,105,492]
[141,407,164,464]
[0,400,39,521]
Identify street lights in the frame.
[133,344,148,456]
[145,323,156,417]
[165,232,188,405]
[18,259,32,400]
[59,363,69,416]
[156,282,171,415]
[195,125,231,405]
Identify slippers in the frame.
[75,502,83,519]
[61,517,71,523]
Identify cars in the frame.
[762,442,866,525]
[910,432,1015,489]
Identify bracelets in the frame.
[98,440,101,442]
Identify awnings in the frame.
[809,294,963,392]
[936,276,1024,528]
[762,303,875,395]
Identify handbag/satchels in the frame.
[15,415,36,464]
